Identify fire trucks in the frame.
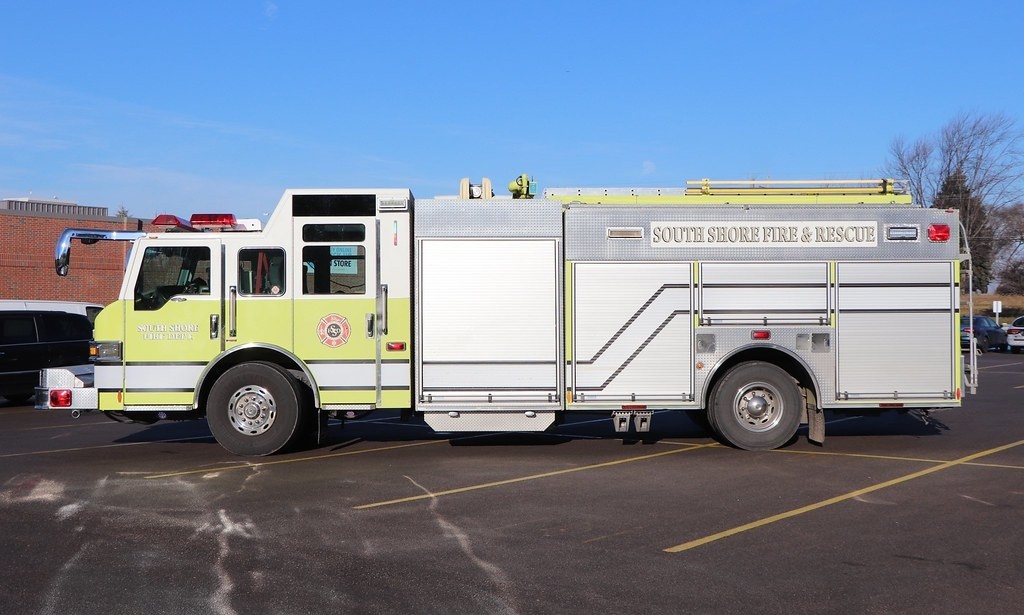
[40,174,977,452]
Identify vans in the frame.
[0,309,94,402]
[1,299,106,329]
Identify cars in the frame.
[961,314,1008,352]
[1006,315,1024,352]
